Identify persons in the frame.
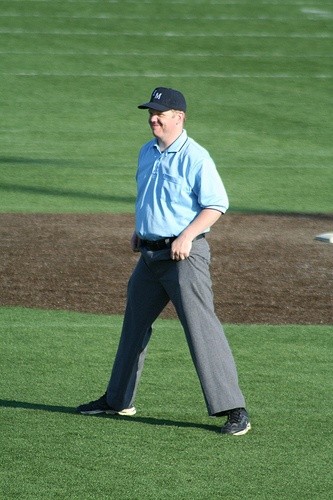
[77,88,253,437]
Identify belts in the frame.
[139,232,205,251]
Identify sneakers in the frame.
[77,391,136,415]
[219,407,252,436]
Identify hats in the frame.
[138,86,186,113]
